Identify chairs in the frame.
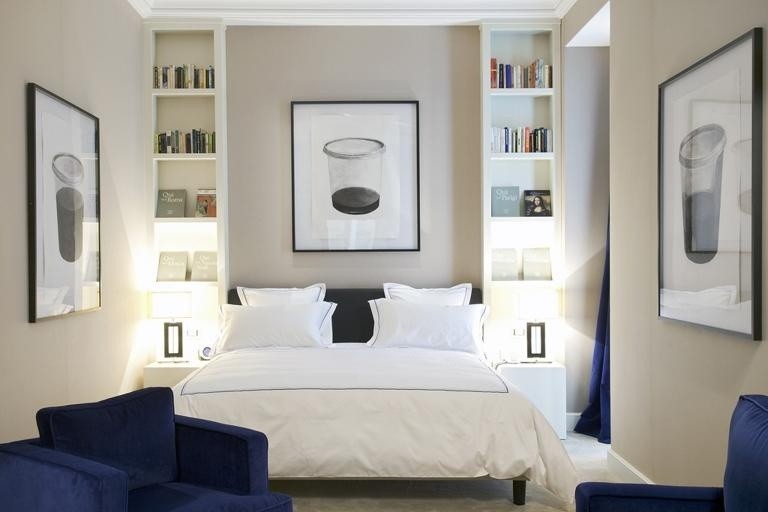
[0,388,292,512]
[575,393,767,509]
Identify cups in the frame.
[678,123,728,266]
[50,150,87,265]
[323,137,387,215]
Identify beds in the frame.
[166,287,579,505]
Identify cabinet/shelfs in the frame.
[479,19,563,322]
[143,17,227,308]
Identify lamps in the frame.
[514,289,561,366]
[149,293,196,363]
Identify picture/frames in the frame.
[290,99,422,253]
[658,27,762,341]
[26,83,102,325]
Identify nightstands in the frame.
[142,363,203,393]
[497,365,566,440]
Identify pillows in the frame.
[237,282,329,306]
[383,282,473,305]
[210,302,339,358]
[366,298,493,368]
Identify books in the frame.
[152,64,217,282]
[489,58,553,282]
